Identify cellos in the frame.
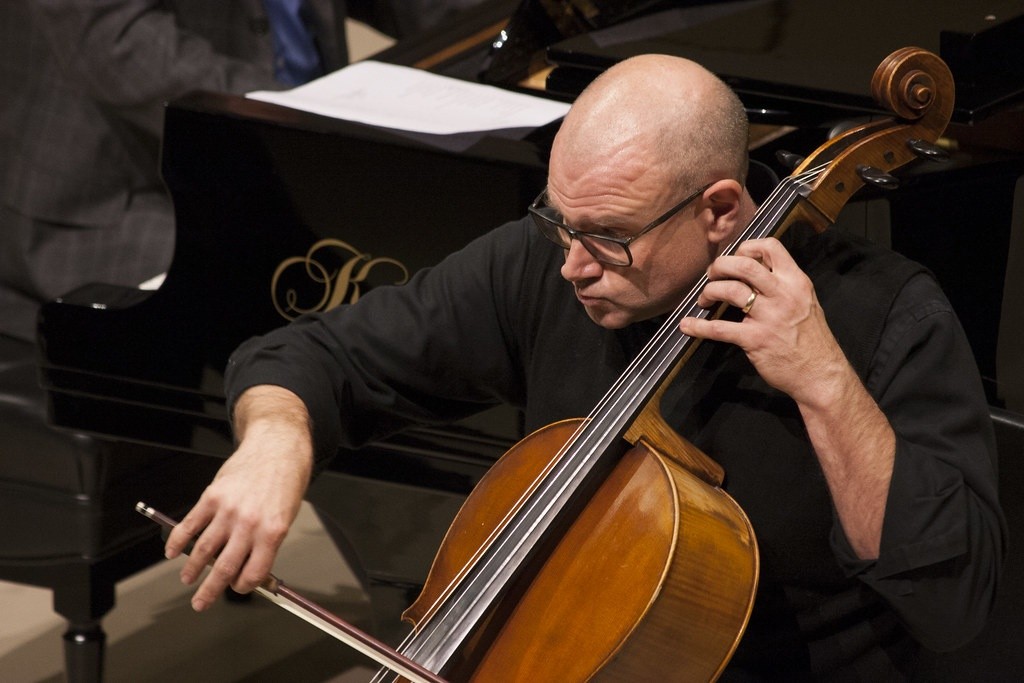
[361,44,959,683]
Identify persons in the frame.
[0,0,501,356]
[165,51,1008,681]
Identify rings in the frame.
[743,289,759,314]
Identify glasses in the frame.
[527,182,718,267]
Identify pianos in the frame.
[34,1,1023,683]
